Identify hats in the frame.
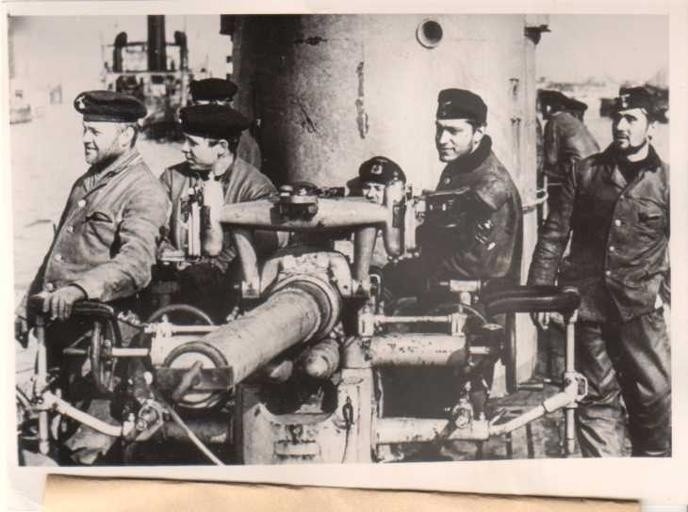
[358,155,407,186]
[538,88,589,113]
[73,90,148,122]
[190,78,238,100]
[613,87,657,121]
[179,106,252,140]
[437,88,488,121]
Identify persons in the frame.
[359,156,419,268]
[13,79,305,457]
[414,88,522,420]
[521,85,672,456]
